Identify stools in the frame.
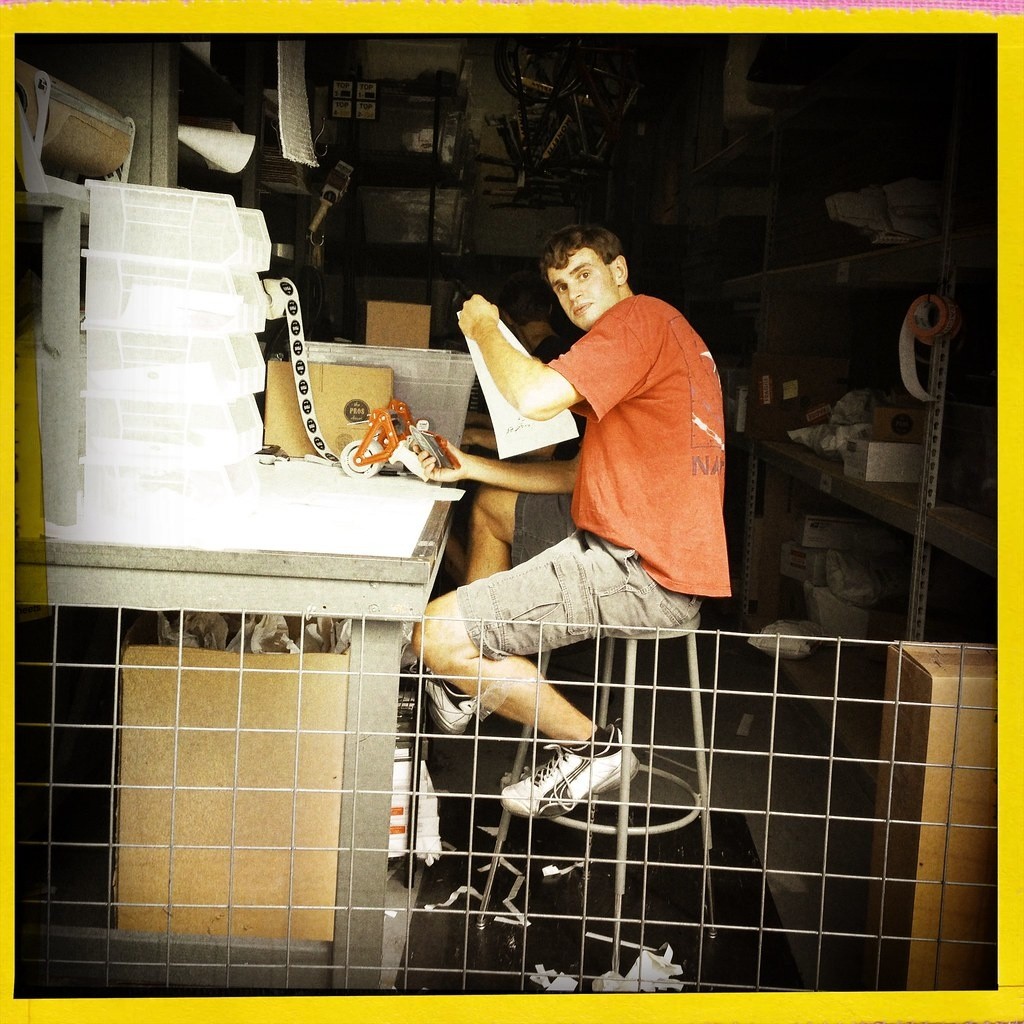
[474,612,720,941]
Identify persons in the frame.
[395,224,733,821]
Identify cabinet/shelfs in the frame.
[689,40,999,788]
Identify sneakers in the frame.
[408,658,473,735]
[500,721,641,820]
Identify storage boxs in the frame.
[78,178,272,525]
[303,339,477,447]
[856,641,999,991]
[110,610,351,943]
[264,362,394,460]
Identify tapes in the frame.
[908,295,962,346]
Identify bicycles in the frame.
[482,34,661,207]
